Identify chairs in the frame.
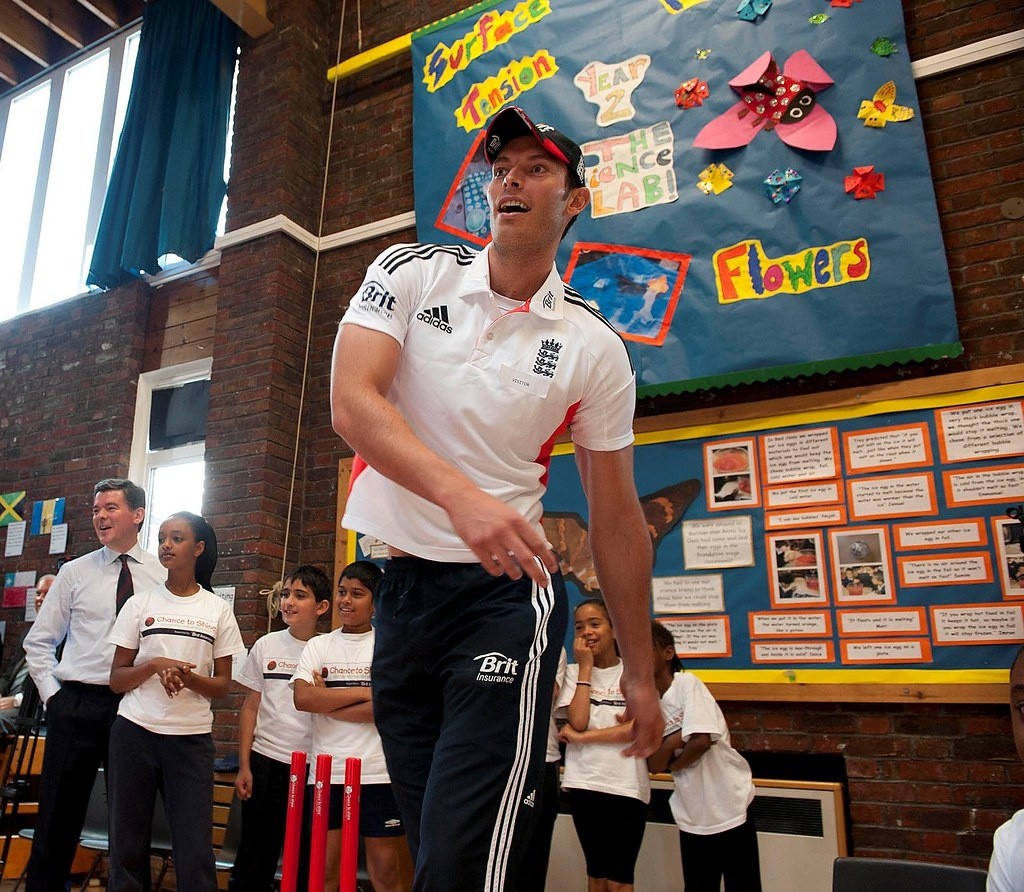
[80,784,183,889]
[13,768,115,891]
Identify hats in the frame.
[483,106,585,187]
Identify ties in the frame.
[116,554,134,620]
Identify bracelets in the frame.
[576,681,591,686]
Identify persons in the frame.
[332,105,664,892]
[1,478,250,892]
[237,560,763,892]
[984,645,1024,892]
